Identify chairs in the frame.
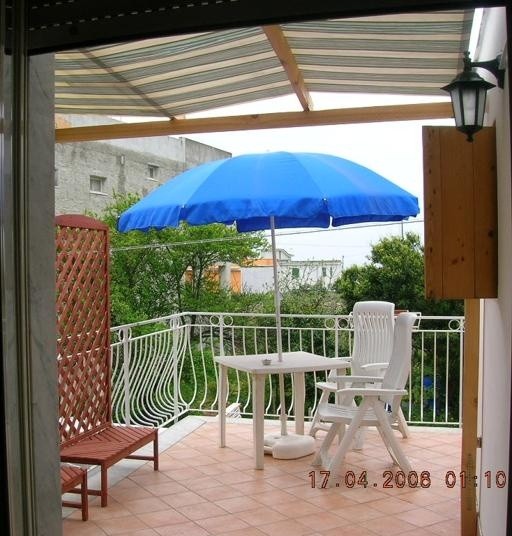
[310,300,410,447]
[311,313,419,488]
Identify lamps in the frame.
[441,49,505,142]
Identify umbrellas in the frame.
[117,151,421,435]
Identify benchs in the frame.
[61,466,89,522]
[61,425,158,506]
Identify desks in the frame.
[214,351,351,470]
